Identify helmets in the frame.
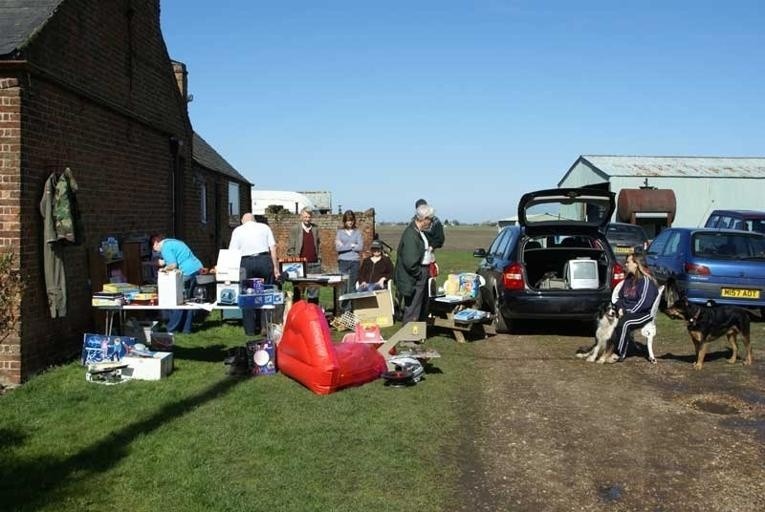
[381,354,425,388]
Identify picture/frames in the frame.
[279,261,306,279]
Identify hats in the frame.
[369,240,383,249]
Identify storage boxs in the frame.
[215,248,247,307]
[121,352,174,381]
[121,320,158,345]
[352,290,393,328]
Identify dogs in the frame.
[663,295,765,370]
[575,300,643,364]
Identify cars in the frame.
[474,181,626,335]
[644,227,765,318]
[704,210,765,231]
[604,222,650,263]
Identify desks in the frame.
[273,273,348,317]
[429,299,496,343]
[98,304,276,341]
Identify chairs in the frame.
[354,278,395,318]
[277,301,385,396]
[611,280,665,366]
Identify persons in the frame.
[230,213,280,336]
[393,204,434,326]
[354,239,393,292]
[607,254,659,366]
[334,210,363,313]
[286,206,321,306]
[415,199,445,248]
[149,235,203,299]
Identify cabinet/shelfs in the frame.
[88,241,151,334]
